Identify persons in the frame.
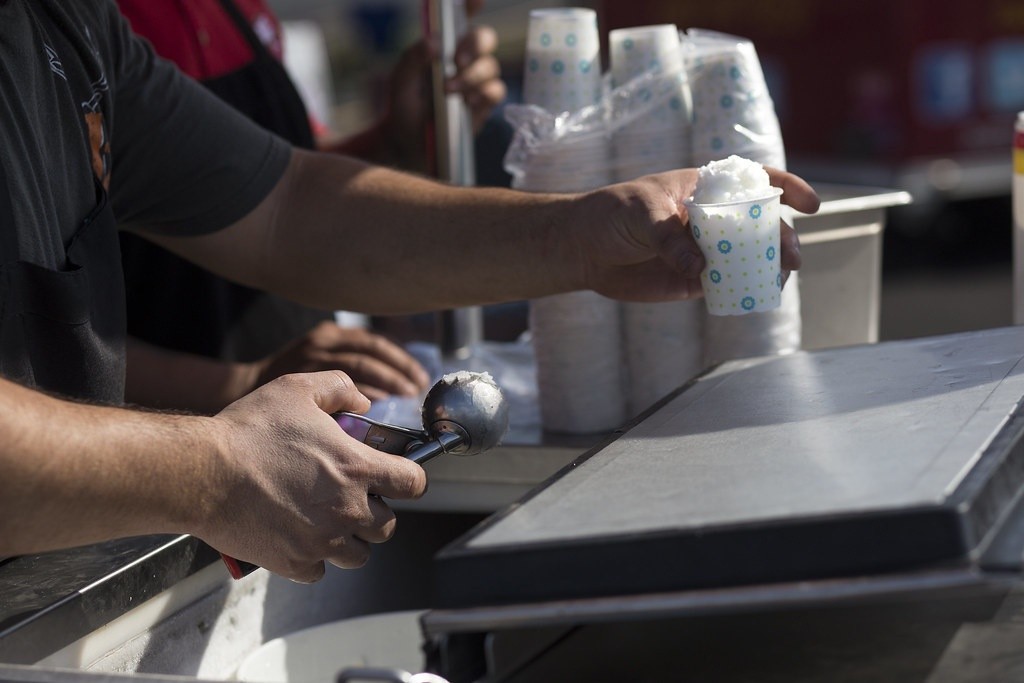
[0,0,821,586]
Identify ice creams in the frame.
[681,153,781,317]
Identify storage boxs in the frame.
[792,183,913,355]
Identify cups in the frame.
[521,9,802,433]
